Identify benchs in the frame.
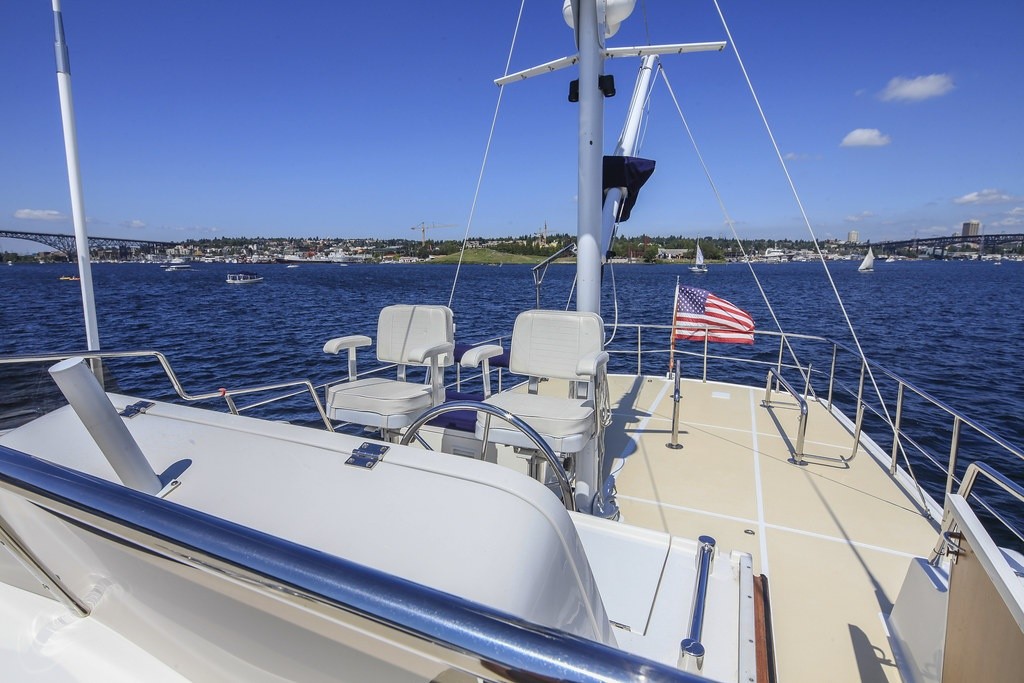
[384,343,546,486]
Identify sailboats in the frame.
[688,239,708,272]
[858,247,874,272]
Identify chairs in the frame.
[323,304,454,444]
[458,310,608,482]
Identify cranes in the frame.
[410,220,458,246]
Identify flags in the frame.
[673,285,755,345]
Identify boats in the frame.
[287,264,298,268]
[59,275,80,281]
[886,258,895,263]
[225,274,263,283]
[165,265,199,272]
[340,264,348,266]
[160,265,168,268]
